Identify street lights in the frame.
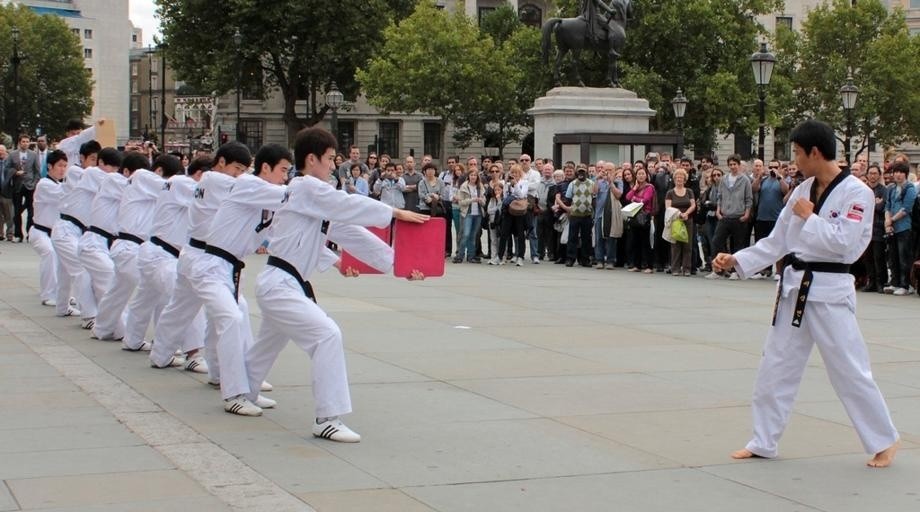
[671,89,688,148]
[158,43,168,153]
[10,26,23,143]
[840,74,858,164]
[326,81,342,140]
[231,28,243,138]
[749,43,775,163]
[145,45,154,126]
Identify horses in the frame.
[542,0,633,87]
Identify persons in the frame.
[709,122,902,469]
[285,146,807,280]
[243,127,432,444]
[838,150,920,295]
[0,132,275,395]
[189,143,359,417]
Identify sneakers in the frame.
[468,252,483,263]
[750,273,768,279]
[628,266,664,273]
[312,418,361,443]
[862,285,915,295]
[123,341,152,350]
[185,357,210,373]
[532,256,543,264]
[597,262,624,269]
[451,259,462,263]
[483,256,525,266]
[212,380,276,415]
[82,319,97,328]
[151,357,184,368]
[705,272,725,279]
[666,261,702,276]
[41,296,82,317]
[729,272,738,279]
[0,237,21,243]
[550,255,592,267]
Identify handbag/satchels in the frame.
[509,199,527,215]
[672,220,689,243]
[432,204,445,216]
[620,203,649,227]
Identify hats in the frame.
[576,164,587,173]
